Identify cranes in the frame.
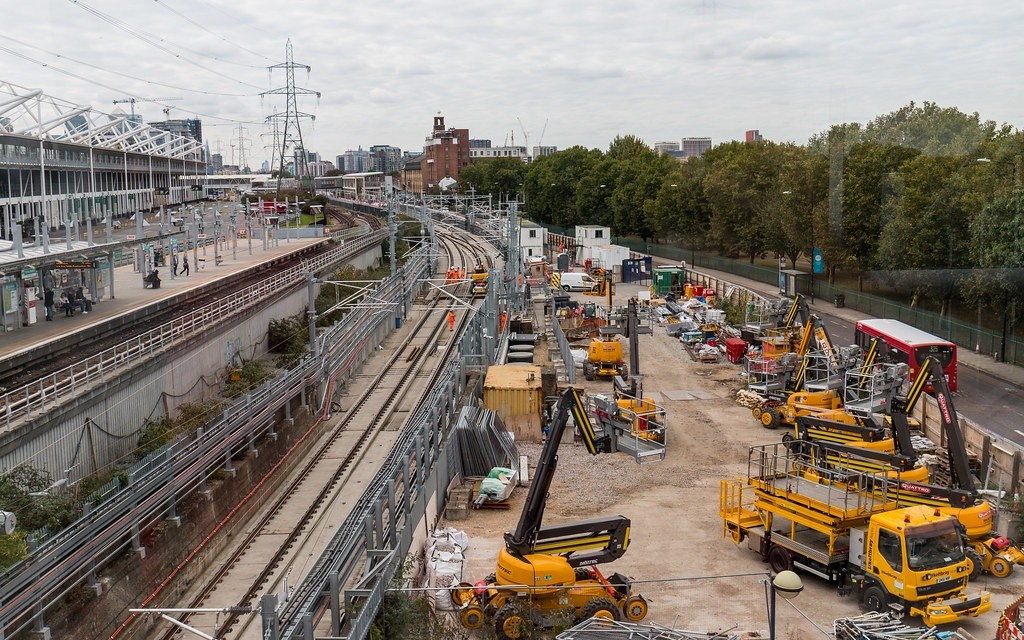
[161,104,176,123]
[113,97,184,143]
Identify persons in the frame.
[59,292,75,319]
[500,310,507,332]
[179,252,190,276]
[151,269,161,289]
[76,287,93,311]
[173,253,179,276]
[445,266,464,286]
[517,271,521,285]
[67,290,88,315]
[45,287,56,322]
[448,309,455,331]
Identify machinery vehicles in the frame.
[583,271,628,381]
[805,356,1023,577]
[614,300,666,443]
[451,385,648,640]
[752,293,900,455]
[719,440,992,628]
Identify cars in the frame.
[473,283,486,294]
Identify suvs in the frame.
[744,343,776,379]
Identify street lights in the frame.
[519,181,530,221]
[783,191,814,305]
[671,184,695,267]
[600,183,619,245]
[977,157,1016,363]
[551,183,568,230]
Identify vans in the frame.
[561,273,598,292]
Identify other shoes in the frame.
[179,273,191,277]
[65,314,76,317]
[45,316,53,322]
[81,311,89,315]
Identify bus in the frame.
[855,318,958,395]
